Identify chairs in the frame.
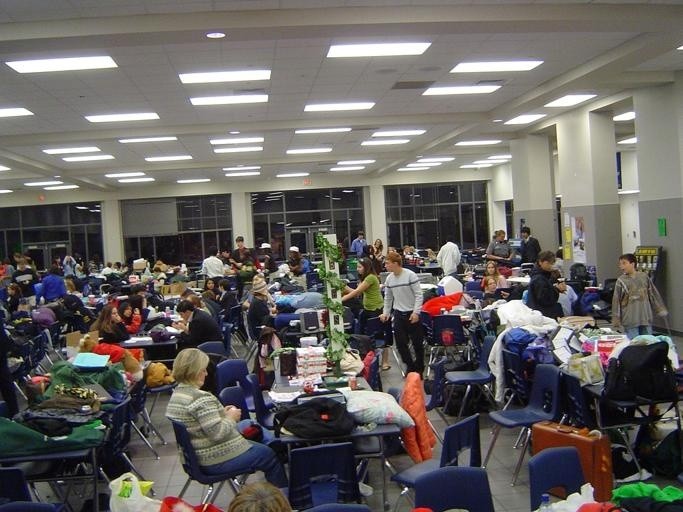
[0,244,682,511]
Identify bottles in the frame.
[153,304,177,318]
[539,493,554,512]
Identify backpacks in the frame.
[639,430,682,480]
[441,380,481,422]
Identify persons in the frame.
[1,237,313,348]
[229,482,291,512]
[482,227,578,319]
[612,254,669,339]
[437,236,461,276]
[342,231,424,380]
[166,349,289,488]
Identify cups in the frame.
[303,380,314,394]
[88,294,94,304]
[348,377,357,390]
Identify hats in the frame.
[259,243,271,249]
[289,246,299,252]
[252,275,267,296]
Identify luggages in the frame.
[531,420,614,503]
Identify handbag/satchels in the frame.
[339,348,364,375]
[570,354,605,388]
[273,389,355,438]
[108,473,160,512]
[600,341,678,403]
